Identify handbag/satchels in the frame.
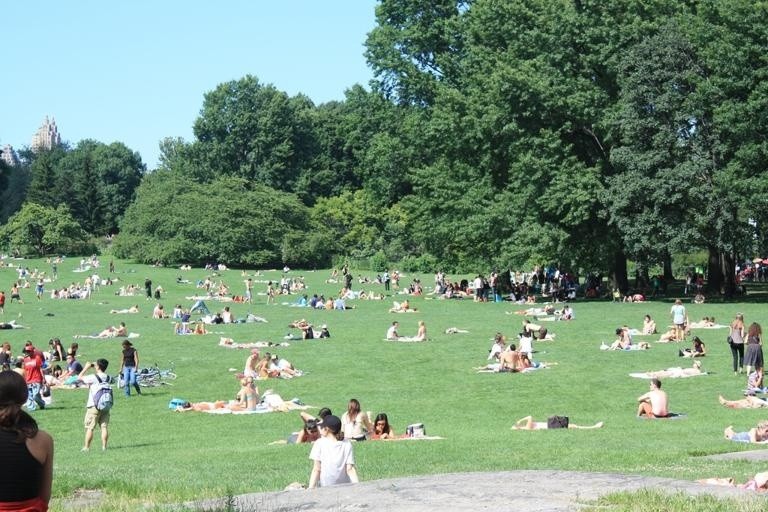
[43,383,50,397]
[548,416,568,428]
[728,337,733,344]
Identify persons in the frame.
[0,253,112,313]
[0,338,139,451]
[174,264,233,335]
[230,350,394,444]
[293,320,328,340]
[671,299,768,409]
[488,305,574,372]
[1,370,54,510]
[100,277,168,337]
[387,321,426,341]
[586,269,702,303]
[435,265,576,303]
[299,268,422,313]
[725,419,768,489]
[513,416,603,430]
[232,266,305,323]
[612,314,700,418]
[737,265,768,283]
[309,415,359,489]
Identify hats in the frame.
[316,415,341,431]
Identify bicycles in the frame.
[136,362,177,387]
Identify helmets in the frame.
[25,346,33,353]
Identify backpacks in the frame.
[92,374,113,411]
